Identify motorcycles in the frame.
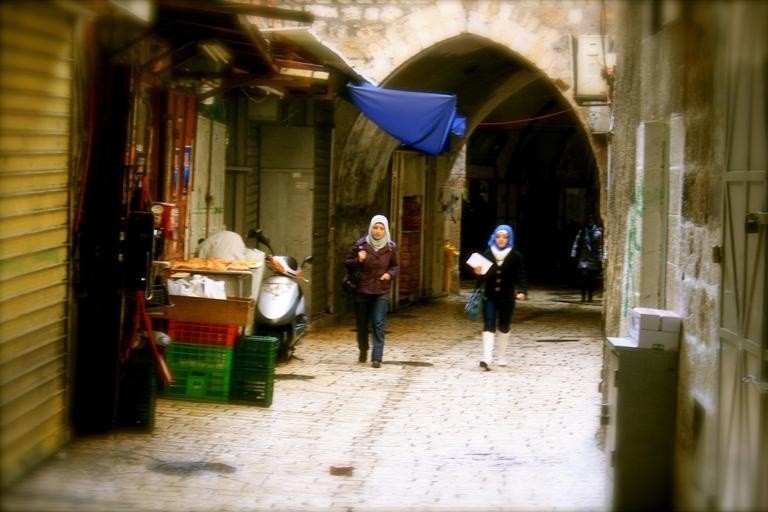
[245,227,314,365]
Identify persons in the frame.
[570,211,602,302]
[470,222,529,372]
[342,213,401,370]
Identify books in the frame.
[464,250,495,277]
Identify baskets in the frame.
[232,337,280,409]
[164,343,232,375]
[168,318,238,347]
[158,372,231,406]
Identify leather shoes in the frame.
[373,361,380,368]
[359,352,368,362]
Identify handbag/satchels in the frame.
[342,242,363,296]
[464,280,486,321]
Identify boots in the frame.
[480,331,495,370]
[497,330,511,365]
